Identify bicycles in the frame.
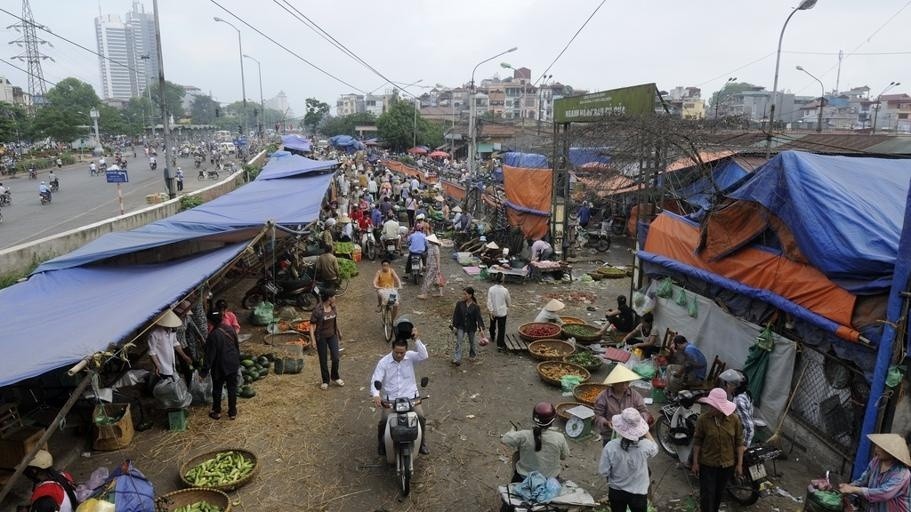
[301,261,348,298]
[803,466,872,511]
[376,288,396,341]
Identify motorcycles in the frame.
[576,227,610,252]
[198,168,218,181]
[410,253,424,284]
[150,159,157,170]
[49,181,59,191]
[0,190,12,207]
[374,378,430,495]
[242,276,320,314]
[361,226,399,259]
[39,190,52,205]
[654,390,780,505]
[91,161,126,175]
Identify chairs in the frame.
[657,327,678,354]
[688,354,726,392]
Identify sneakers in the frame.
[420,447,430,455]
[209,413,220,419]
[228,414,236,419]
[378,446,385,455]
[321,383,328,390]
[335,379,344,386]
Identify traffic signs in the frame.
[106,170,128,183]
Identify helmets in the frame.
[532,402,556,426]
[719,369,748,387]
[393,318,414,339]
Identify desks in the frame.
[531,263,573,282]
[493,265,528,284]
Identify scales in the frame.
[565,406,595,443]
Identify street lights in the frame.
[872,79,902,137]
[766,0,819,160]
[214,16,245,131]
[468,47,519,174]
[797,65,824,130]
[502,62,527,117]
[538,74,551,137]
[395,79,422,101]
[715,77,736,118]
[243,54,265,137]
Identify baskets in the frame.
[417,294,427,299]
[451,359,460,366]
[178,448,258,492]
[153,488,230,512]
[562,353,603,371]
[559,317,585,325]
[597,267,626,278]
[561,323,603,341]
[289,319,316,335]
[572,383,610,404]
[264,332,311,349]
[554,402,595,420]
[518,322,562,341]
[536,361,591,386]
[528,339,575,361]
[432,293,443,297]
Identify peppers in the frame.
[174,500,221,512]
[185,451,254,487]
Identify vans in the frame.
[220,142,234,153]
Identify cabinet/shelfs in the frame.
[0,426,49,469]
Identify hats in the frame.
[25,451,53,470]
[339,216,351,223]
[486,241,499,249]
[698,388,736,416]
[545,299,565,311]
[152,309,182,328]
[452,206,462,213]
[611,408,649,442]
[425,234,440,244]
[175,300,193,315]
[866,433,911,467]
[604,364,640,384]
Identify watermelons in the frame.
[239,351,271,399]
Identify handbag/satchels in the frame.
[436,273,446,287]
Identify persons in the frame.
[533,293,708,400]
[309,246,403,390]
[500,359,757,512]
[1,128,247,201]
[310,139,512,279]
[838,433,911,512]
[415,233,444,300]
[528,239,554,262]
[576,200,591,229]
[0,284,240,511]
[371,327,431,466]
[448,270,512,366]
